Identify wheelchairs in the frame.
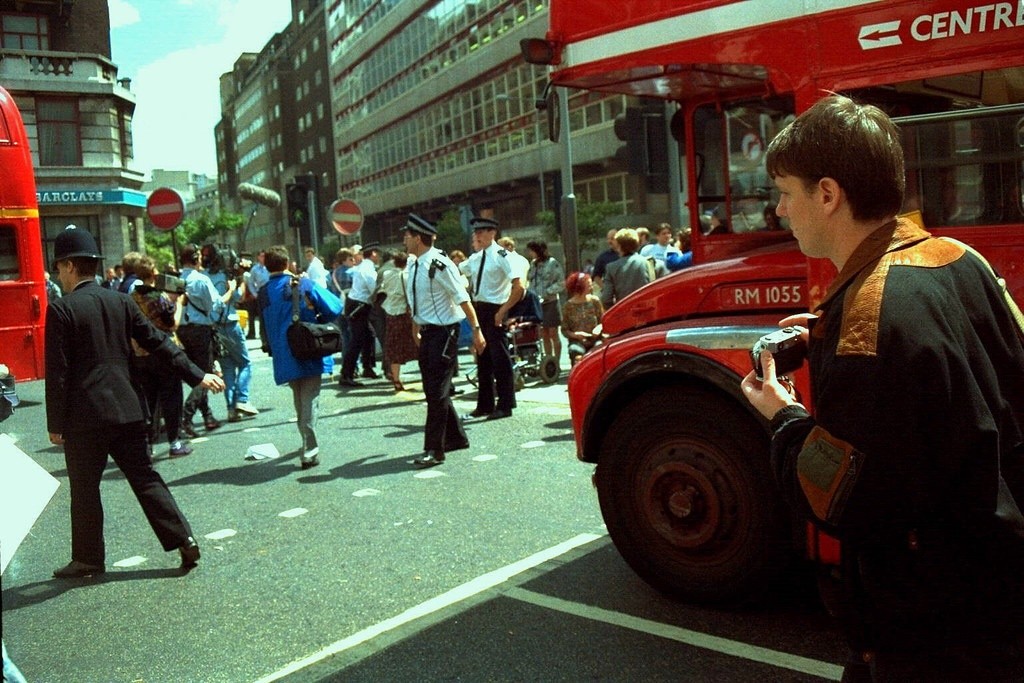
[465,318,561,390]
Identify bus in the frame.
[0,84,48,383]
[516,1,1024,615]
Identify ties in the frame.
[412,259,419,317]
[474,250,486,296]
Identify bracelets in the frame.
[471,325,481,330]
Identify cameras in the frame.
[751,326,805,380]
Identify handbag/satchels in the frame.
[288,317,344,360]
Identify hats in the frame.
[358,240,381,251]
[469,217,499,230]
[51,228,107,260]
[399,212,437,235]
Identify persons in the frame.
[759,205,784,230]
[739,89,1024,683]
[34,202,735,469]
[43,225,226,579]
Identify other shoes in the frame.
[179,536,200,567]
[206,419,225,430]
[360,369,383,380]
[444,442,470,451]
[387,373,404,390]
[336,376,365,388]
[54,560,106,577]
[414,454,445,465]
[228,407,243,420]
[235,400,259,416]
[301,459,321,470]
[470,407,512,419]
[168,444,193,458]
[180,418,200,437]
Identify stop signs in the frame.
[328,198,364,237]
[146,185,183,232]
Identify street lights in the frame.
[497,93,548,244]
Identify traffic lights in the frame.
[613,106,646,177]
[285,182,309,227]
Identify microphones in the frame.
[238,183,281,209]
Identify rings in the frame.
[49,438,52,442]
[210,381,214,385]
